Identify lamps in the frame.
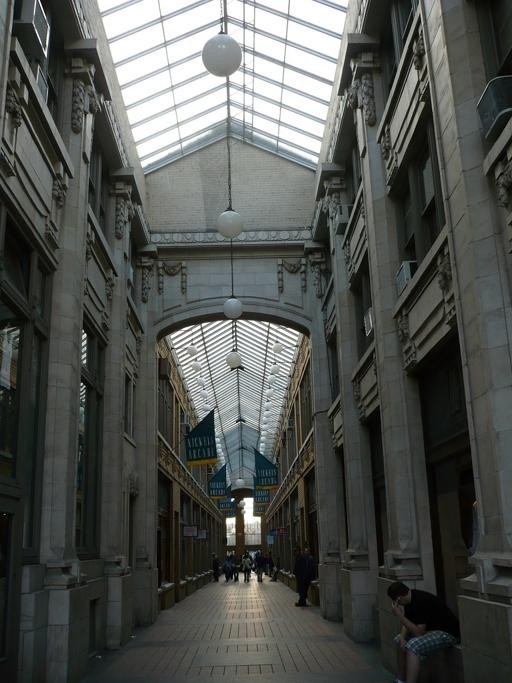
[257,339,299,441]
[199,0,243,79]
[168,343,213,412]
[213,116,249,369]
[233,446,247,487]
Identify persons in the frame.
[387,581,461,683]
[302,546,316,599]
[210,548,281,582]
[291,545,308,608]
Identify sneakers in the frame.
[212,573,277,583]
[295,599,307,607]
